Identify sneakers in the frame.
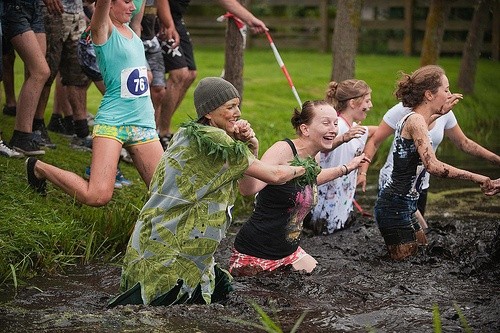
[86,112,95,127]
[69,131,93,153]
[8,130,46,155]
[120,147,134,163]
[32,118,58,153]
[46,114,75,135]
[25,157,48,198]
[0,136,25,160]
[84,165,131,188]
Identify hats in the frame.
[194,77,241,120]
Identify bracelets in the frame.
[356,172,367,177]
[343,165,348,175]
[233,131,242,140]
[342,132,345,142]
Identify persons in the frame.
[303,79,379,236]
[0,0,269,188]
[356,99,500,218]
[25,0,166,207]
[375,64,495,262]
[228,99,371,278]
[107,77,320,306]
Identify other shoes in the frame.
[157,129,174,152]
[3,103,16,116]
